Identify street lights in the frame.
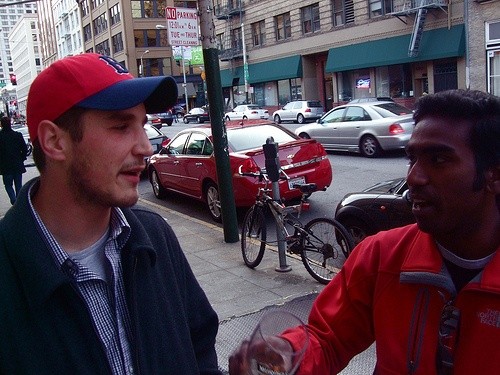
[156,24,190,113]
[141,49,149,77]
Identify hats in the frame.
[26,53,179,143]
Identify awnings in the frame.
[326,23,465,72]
[203,55,302,92]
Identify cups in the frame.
[247,309,309,375]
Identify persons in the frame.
[0,118,27,205]
[0,53,220,375]
[229,88,500,375]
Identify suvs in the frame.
[272,100,326,124]
[174,106,184,118]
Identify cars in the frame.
[140,124,171,180]
[145,114,162,130]
[347,97,395,104]
[183,107,210,124]
[145,114,332,224]
[225,103,270,120]
[333,176,418,260]
[157,112,173,126]
[295,101,420,158]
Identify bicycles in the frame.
[237,164,356,285]
[26,138,33,156]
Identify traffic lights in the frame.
[14,101,17,106]
[11,75,16,82]
